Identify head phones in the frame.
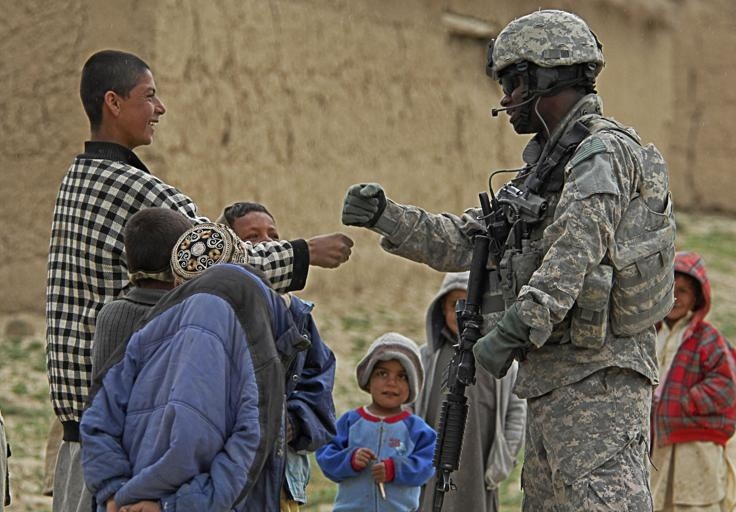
[530,64,560,94]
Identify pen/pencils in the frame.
[371,459,386,500]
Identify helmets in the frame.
[489,7,605,79]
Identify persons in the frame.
[339,8,678,512]
[315,331,439,510]
[647,250,735,512]
[77,263,312,511]
[215,201,338,512]
[88,208,193,391]
[44,47,355,511]
[402,271,526,512]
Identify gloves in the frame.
[339,180,386,229]
[470,302,532,380]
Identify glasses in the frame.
[500,70,524,98]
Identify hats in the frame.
[355,330,426,405]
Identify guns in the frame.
[433,236,492,511]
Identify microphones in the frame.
[492,94,536,117]
